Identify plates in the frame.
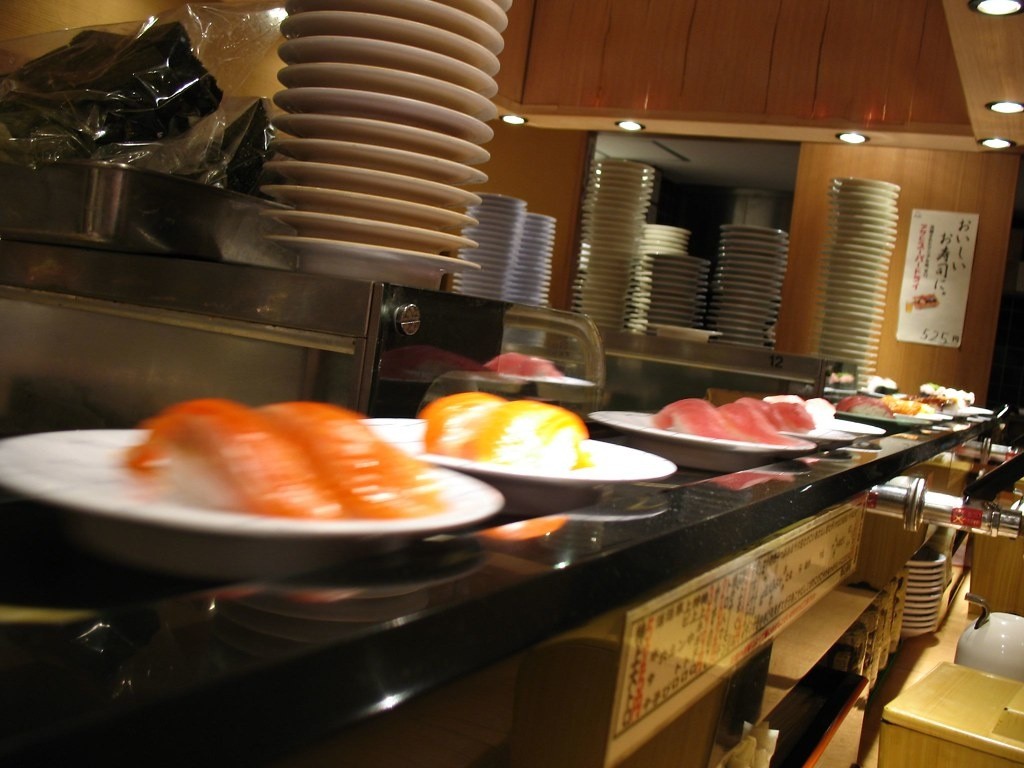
[900,551,945,639]
[589,408,816,473]
[262,0,555,304]
[773,430,854,450]
[365,416,677,516]
[814,178,899,390]
[0,427,504,578]
[816,397,994,437]
[565,156,790,344]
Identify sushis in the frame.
[123,353,976,520]
